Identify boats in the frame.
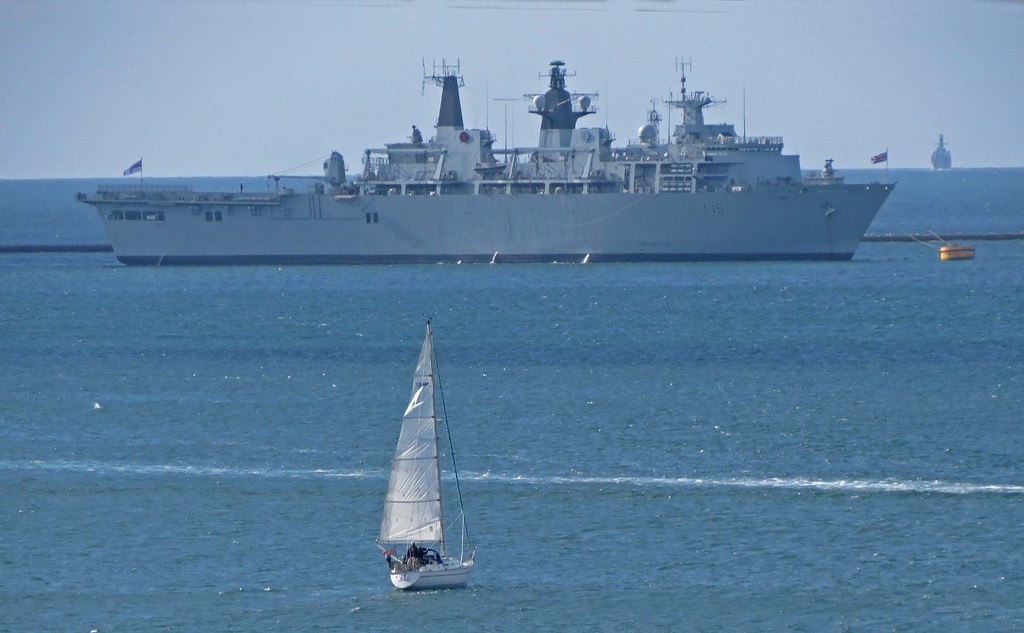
[76,44,897,265]
[931,133,952,169]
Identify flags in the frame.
[123,160,141,176]
[871,151,887,164]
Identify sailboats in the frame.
[375,317,478,590]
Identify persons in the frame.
[407,541,420,558]
